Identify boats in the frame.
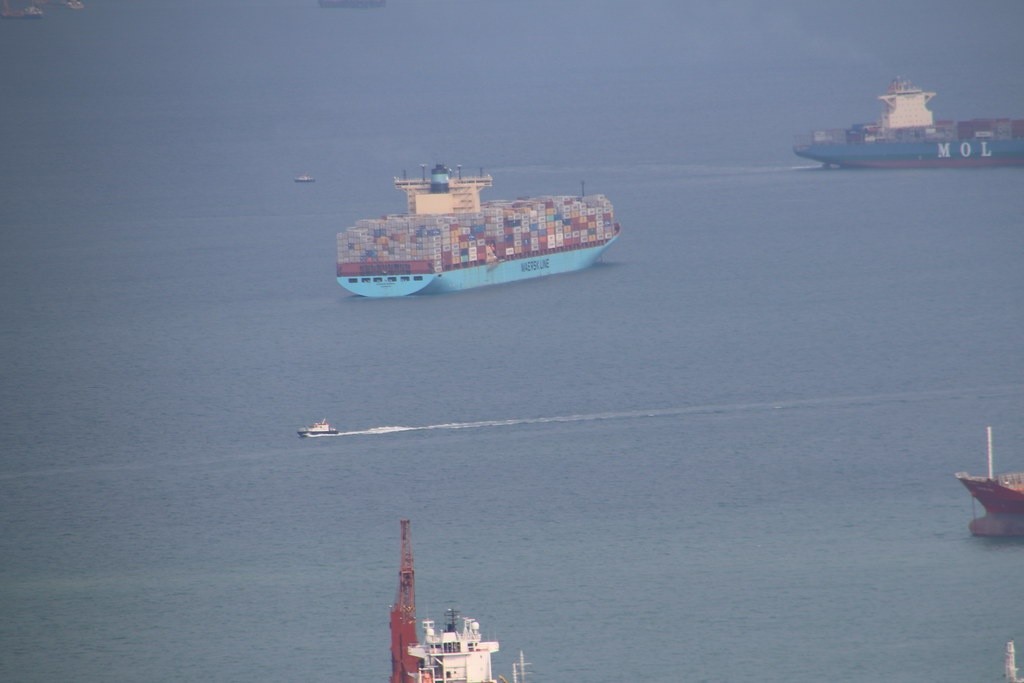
[297,417,339,438]
[952,425,1024,539]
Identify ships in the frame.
[792,73,1024,170]
[335,159,622,298]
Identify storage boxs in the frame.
[338,194,622,276]
